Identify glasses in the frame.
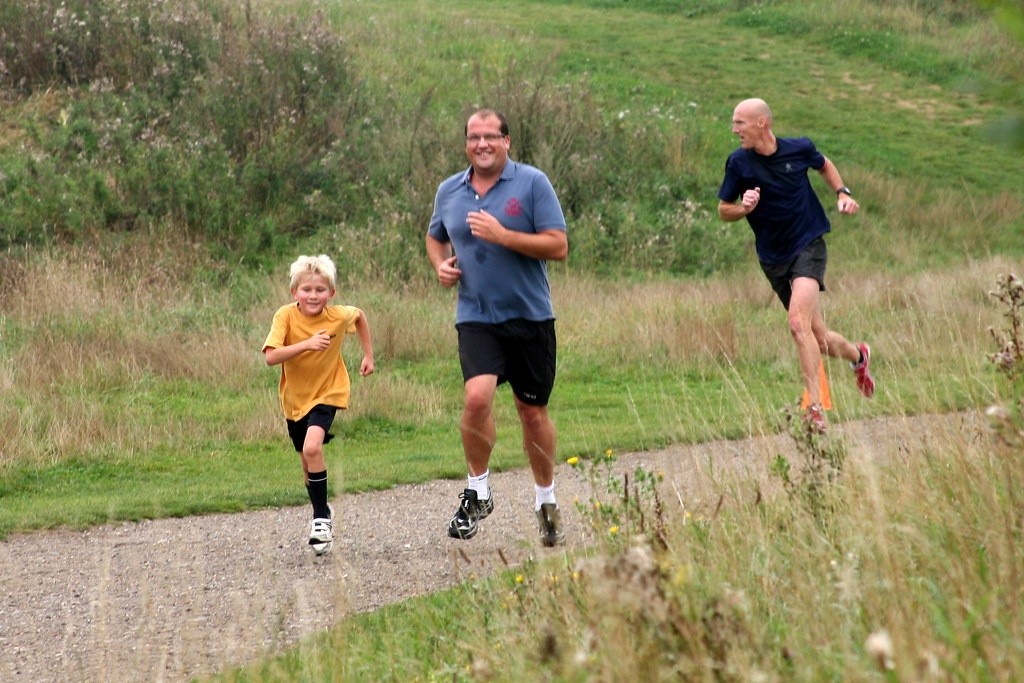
[466,133,505,142]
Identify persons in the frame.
[717,97,875,436]
[426,110,568,547]
[262,255,373,554]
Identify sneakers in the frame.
[850,342,875,398]
[308,506,335,544]
[313,540,332,557]
[535,502,567,547]
[804,403,826,434]
[448,485,495,539]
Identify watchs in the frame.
[837,187,850,198]
